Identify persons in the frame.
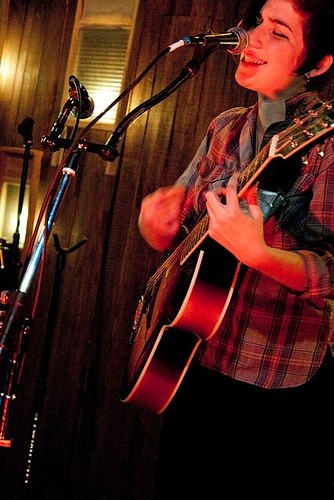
[137,0,334,498]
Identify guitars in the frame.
[122,97,334,414]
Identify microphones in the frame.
[168,27,250,59]
[68,75,95,120]
